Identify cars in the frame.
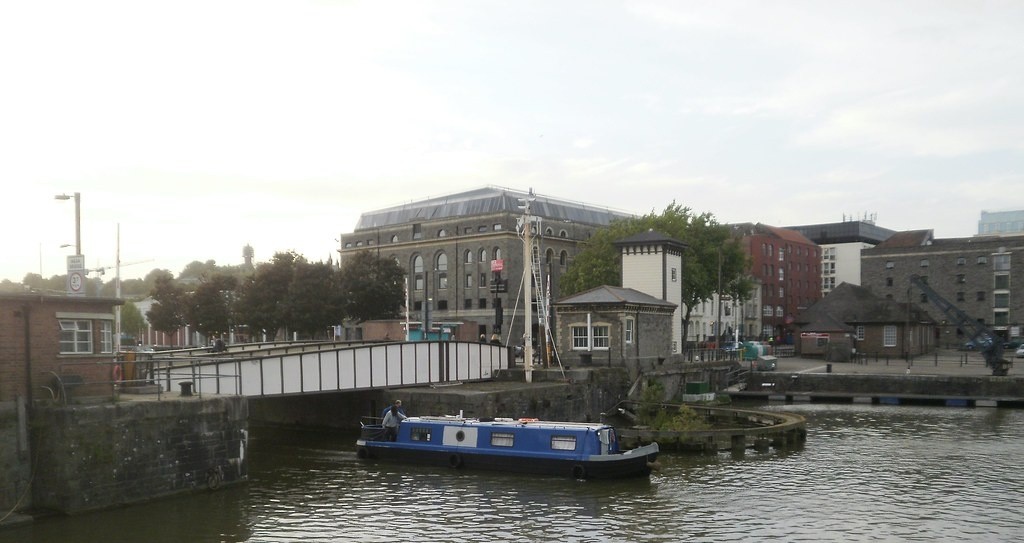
[721,342,743,351]
[1002,338,1024,349]
[513,342,537,358]
[965,338,993,350]
[1016,344,1024,357]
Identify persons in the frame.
[382,406,407,442]
[383,399,407,441]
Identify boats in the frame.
[355,415,659,480]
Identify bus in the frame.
[113,334,141,352]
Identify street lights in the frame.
[54,193,80,256]
